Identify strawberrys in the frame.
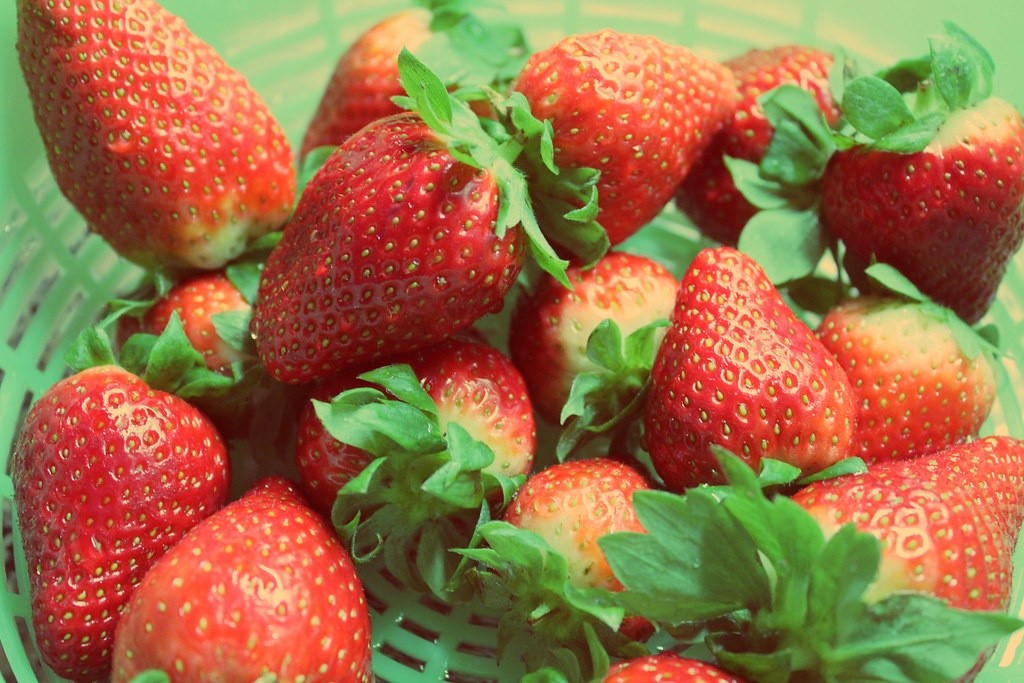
[0,0,1024,683]
